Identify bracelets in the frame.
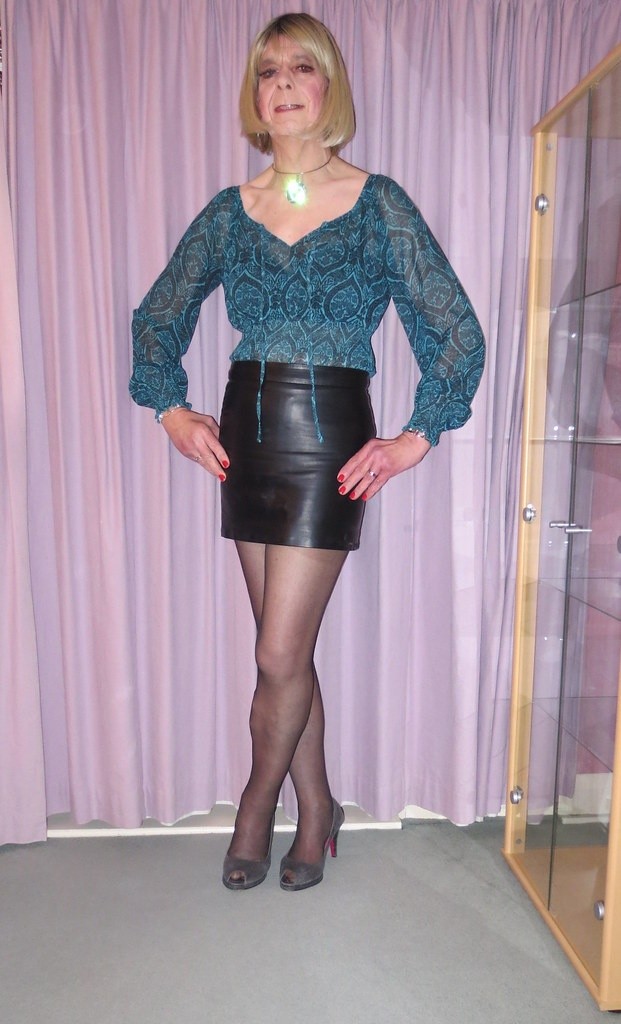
[157,403,182,425]
[407,427,431,442]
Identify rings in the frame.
[368,470,379,479]
[194,455,202,463]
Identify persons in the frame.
[128,13,487,892]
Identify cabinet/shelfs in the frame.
[501,44,621,1012]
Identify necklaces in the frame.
[272,154,334,208]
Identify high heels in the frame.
[279,797,345,892]
[222,813,275,890]
